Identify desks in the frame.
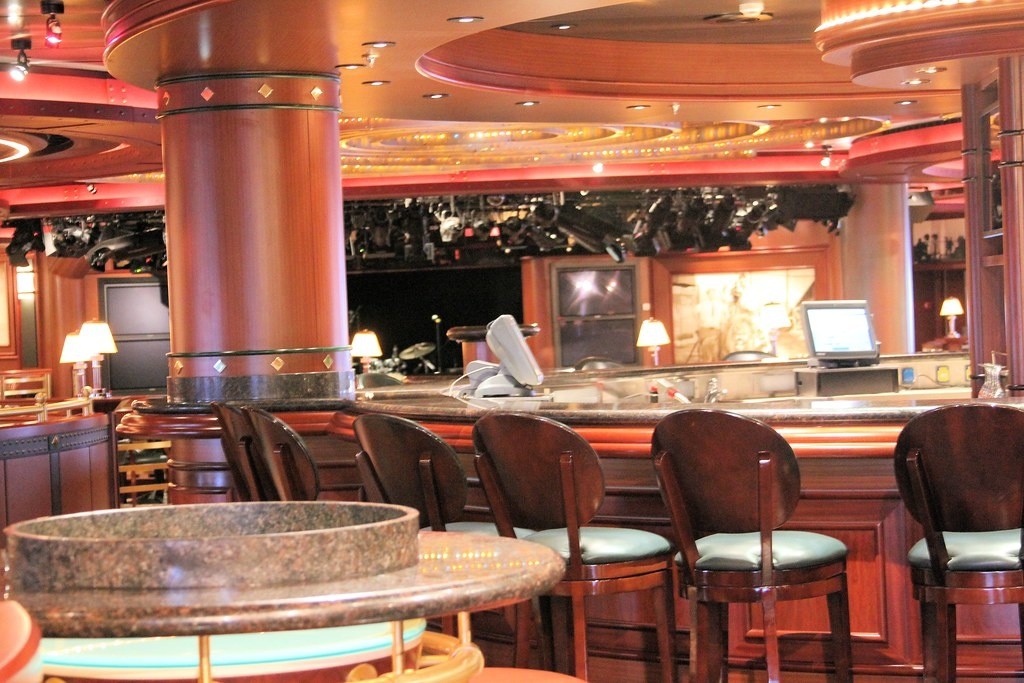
[0,501,567,683]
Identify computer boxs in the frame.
[466,397,554,413]
[794,368,900,397]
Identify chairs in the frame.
[473,413,677,683]
[354,414,541,670]
[649,404,853,683]
[209,399,365,501]
[892,400,1024,683]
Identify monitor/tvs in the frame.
[475,314,544,398]
[802,299,880,368]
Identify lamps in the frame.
[59,331,97,399]
[346,182,855,263]
[351,331,383,372]
[9,36,33,81]
[636,319,671,367]
[3,209,169,306]
[941,297,965,338]
[41,0,66,48]
[77,322,118,392]
